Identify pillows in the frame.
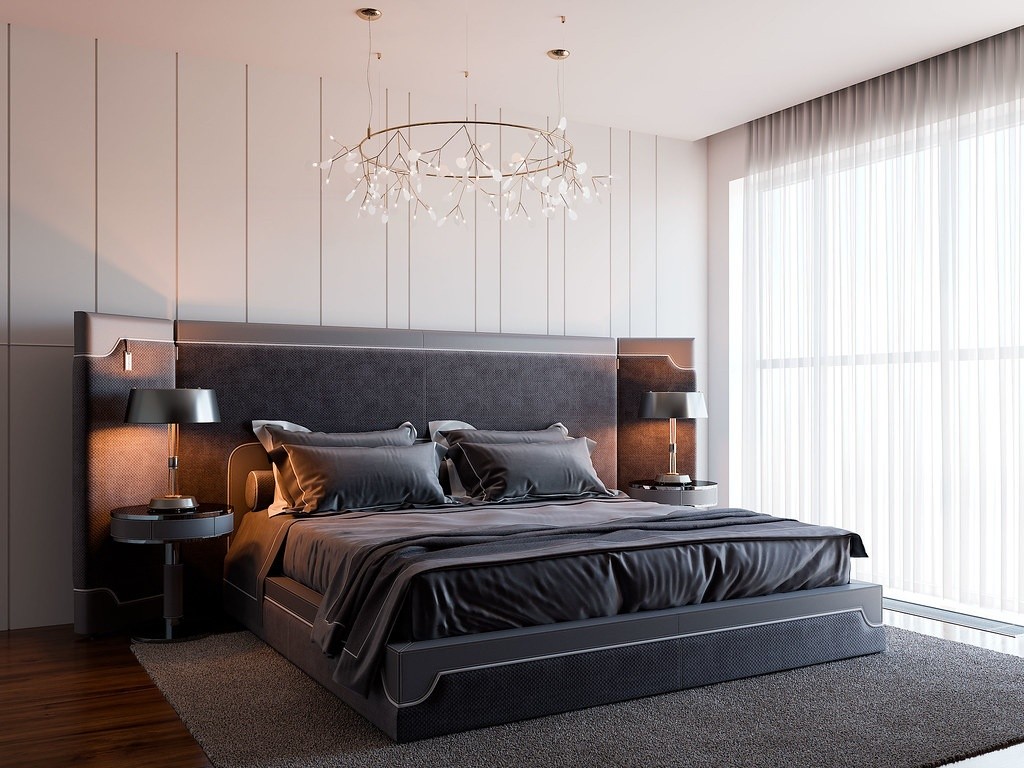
[267,442,462,517]
[441,437,615,506]
[252,420,418,518]
[429,420,575,496]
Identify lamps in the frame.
[312,7,613,226]
[637,390,709,484]
[123,386,222,512]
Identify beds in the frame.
[70,310,887,746]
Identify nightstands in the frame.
[109,504,235,644]
[627,479,718,508]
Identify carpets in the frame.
[128,621,1024,768]
